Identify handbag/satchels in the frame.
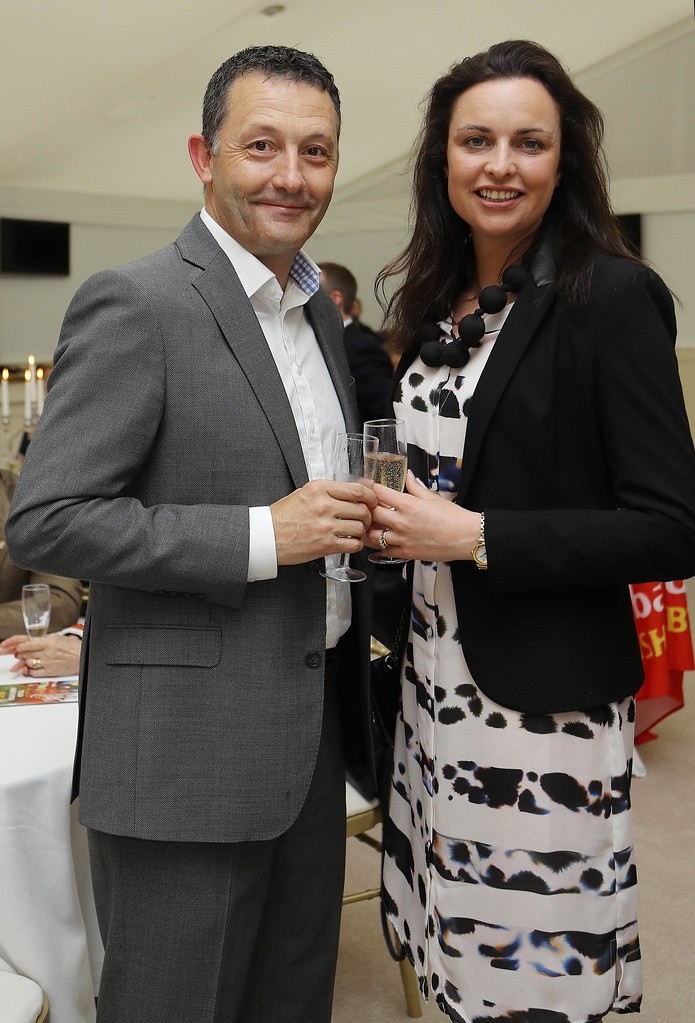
[366,635,404,796]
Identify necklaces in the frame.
[420,252,528,367]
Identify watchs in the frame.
[469,511,488,571]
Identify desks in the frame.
[0,648,105,1022]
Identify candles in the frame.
[2,355,44,421]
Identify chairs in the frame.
[341,643,422,1018]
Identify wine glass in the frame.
[317,431,369,583]
[366,418,414,565]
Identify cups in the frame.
[21,583,52,642]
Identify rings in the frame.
[378,528,390,549]
[32,659,41,669]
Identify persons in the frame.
[370,44,695,1023]
[310,260,404,454]
[0,470,90,680]
[5,44,378,1023]
